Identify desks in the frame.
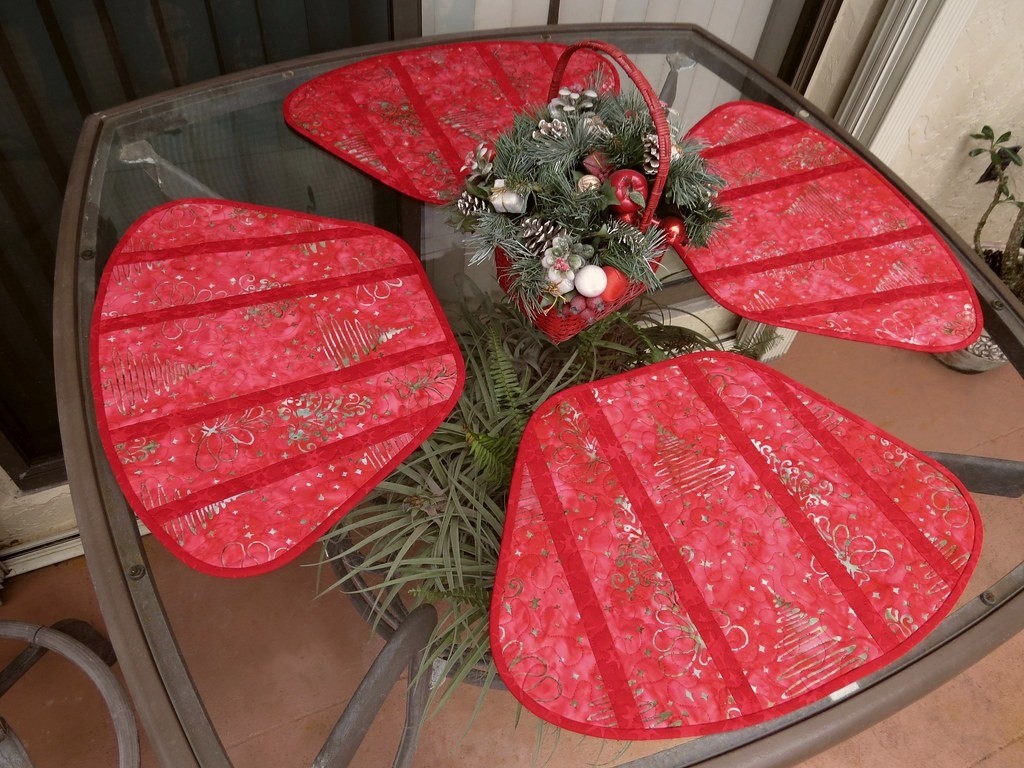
[50,21,1023,768]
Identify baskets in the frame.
[496,39,669,343]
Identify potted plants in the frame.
[925,123,1022,373]
